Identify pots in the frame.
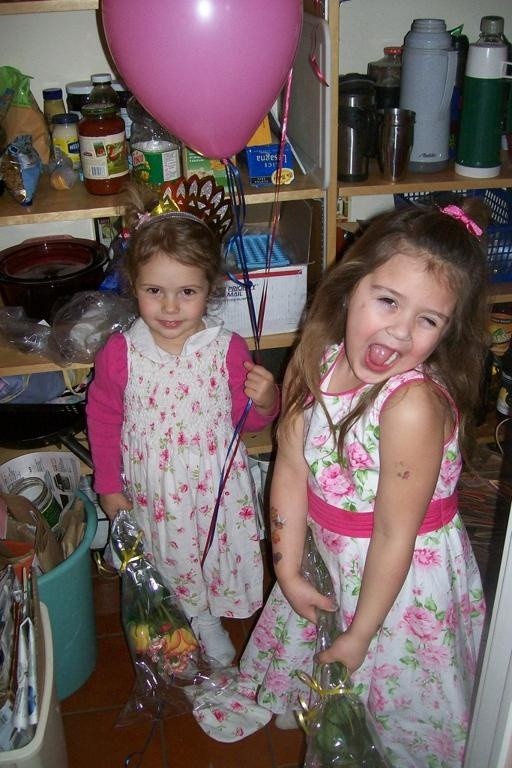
[0,234,112,325]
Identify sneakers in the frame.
[275,709,300,731]
[171,660,199,681]
[197,616,237,670]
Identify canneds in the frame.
[50,80,132,196]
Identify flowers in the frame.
[115,513,204,684]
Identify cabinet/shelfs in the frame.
[331,2,512,447]
[1,0,333,472]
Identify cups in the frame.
[8,477,63,533]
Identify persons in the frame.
[240,202,486,768]
[87,201,284,673]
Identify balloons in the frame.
[91,0,304,168]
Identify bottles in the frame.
[42,86,83,173]
[366,47,405,159]
[86,72,121,109]
[338,72,415,183]
[128,112,182,193]
[449,15,511,180]
[487,302,511,423]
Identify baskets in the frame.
[392,189,511,285]
